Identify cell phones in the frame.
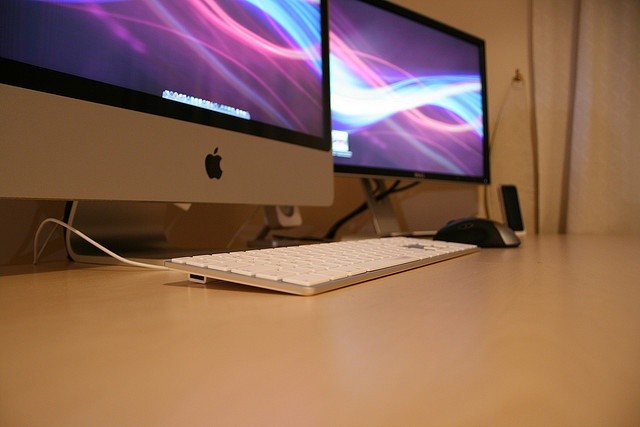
[498,183,527,236]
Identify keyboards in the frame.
[163,235,478,297]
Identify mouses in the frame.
[433,216,521,249]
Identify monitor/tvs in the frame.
[0,1,333,265]
[329,1,491,241]
